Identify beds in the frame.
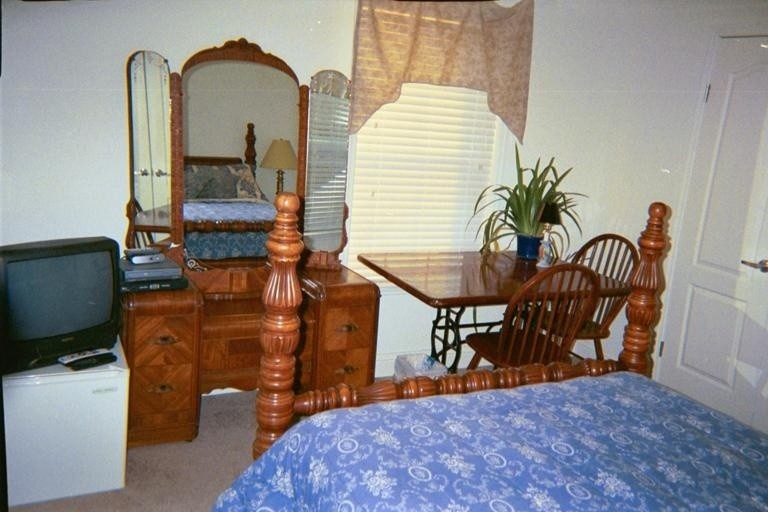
[253,192,768,512]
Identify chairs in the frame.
[466,232,640,372]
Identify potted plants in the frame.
[466,140,592,261]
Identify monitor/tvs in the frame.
[0,234,124,377]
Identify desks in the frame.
[357,251,632,377]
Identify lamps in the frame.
[260,139,298,193]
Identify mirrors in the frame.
[174,36,305,259]
[127,50,173,247]
[306,70,353,270]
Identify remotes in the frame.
[57,347,111,366]
[71,353,119,372]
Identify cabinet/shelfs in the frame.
[2,335,131,507]
[119,267,380,449]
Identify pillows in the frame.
[184,165,268,202]
